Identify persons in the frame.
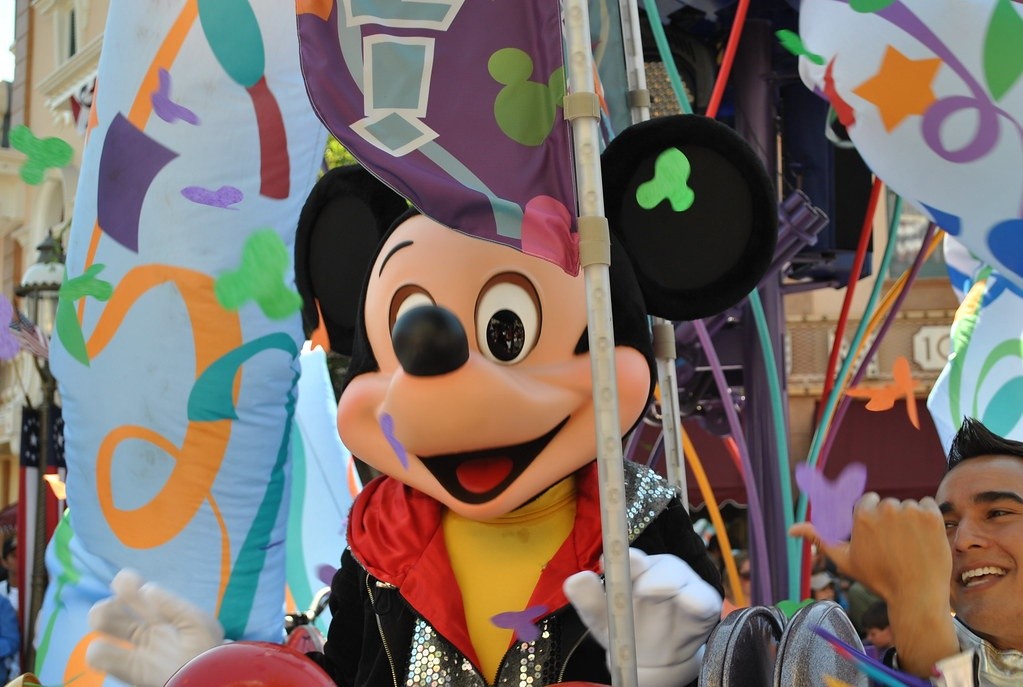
[789,415,1023,684]
[0,533,19,687]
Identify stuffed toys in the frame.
[77,115,782,687]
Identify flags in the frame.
[20,403,68,661]
